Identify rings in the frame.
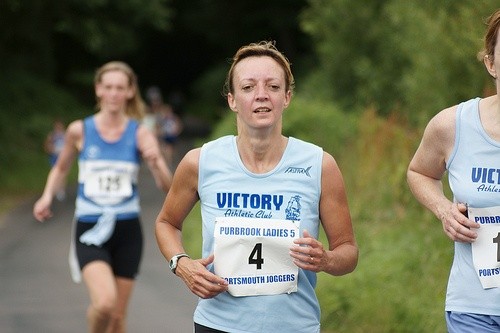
[309,257,316,263]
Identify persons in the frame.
[147,85,179,158]
[154,41,359,333]
[405,9,500,333]
[43,118,71,203]
[33,60,174,333]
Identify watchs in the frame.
[168,254,190,276]
